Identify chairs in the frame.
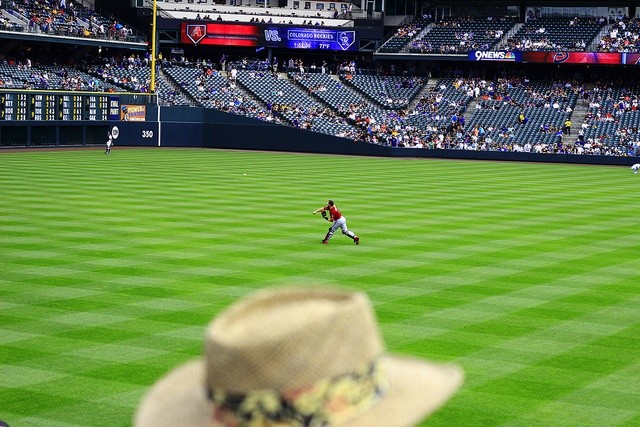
[279,71,465,147]
[188,66,281,126]
[497,17,606,51]
[461,77,581,148]
[606,16,640,55]
[127,64,190,108]
[0,60,128,93]
[410,16,515,52]
[575,82,640,156]
[379,16,410,53]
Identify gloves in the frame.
[321,210,328,220]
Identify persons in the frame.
[287,53,635,156]
[394,10,636,56]
[103,129,116,154]
[312,199,362,247]
[1,1,145,43]
[2,51,288,123]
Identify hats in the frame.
[133,284,468,427]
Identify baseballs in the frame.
[244,173,246,176]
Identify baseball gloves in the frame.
[320,209,326,216]
